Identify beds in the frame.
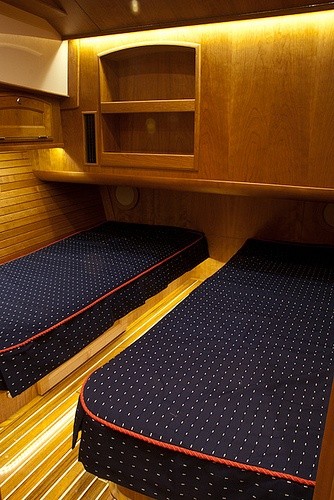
[69,232,332,500]
[0,220,211,398]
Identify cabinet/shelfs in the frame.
[100,43,197,171]
